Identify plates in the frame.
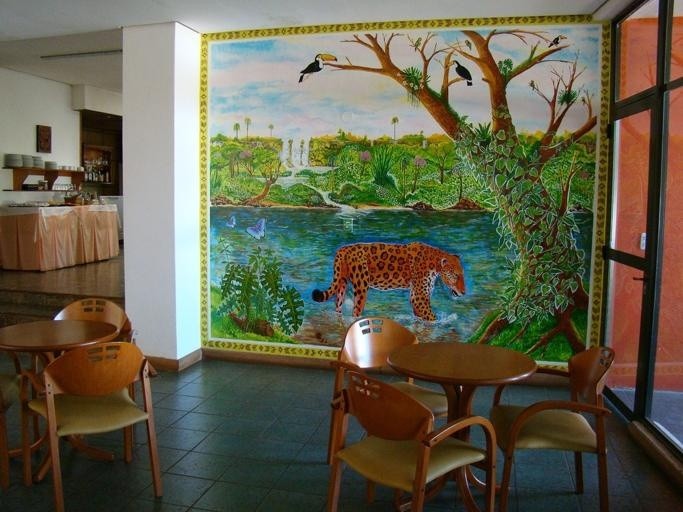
[3,153,58,170]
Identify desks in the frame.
[0,205,121,271]
[386,343,537,511]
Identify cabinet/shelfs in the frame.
[98,195,124,245]
[82,142,113,184]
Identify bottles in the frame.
[84,166,110,183]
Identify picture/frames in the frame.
[37,125,51,153]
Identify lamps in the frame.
[72,85,85,111]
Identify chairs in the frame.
[328,371,495,511]
[0,298,163,511]
[329,317,449,503]
[491,345,615,511]
